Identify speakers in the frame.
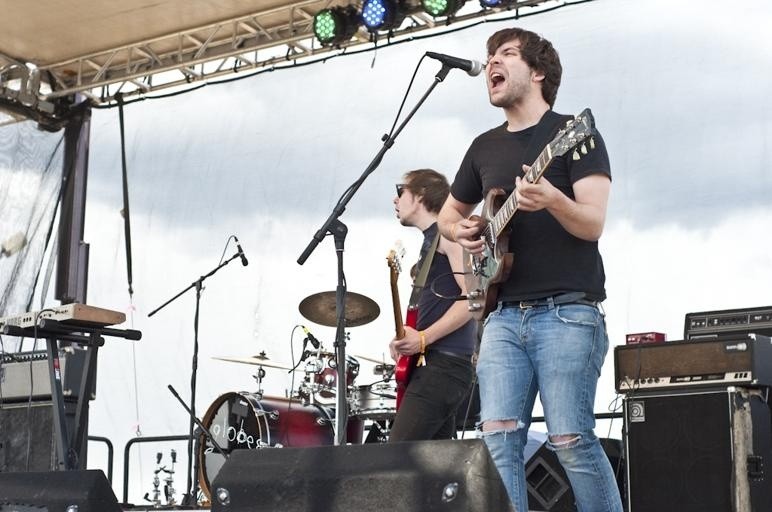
[0,469,122,512]
[210,438,506,512]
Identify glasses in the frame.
[396,184,412,198]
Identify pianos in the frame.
[0,303,127,330]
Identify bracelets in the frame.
[451,221,456,242]
[416,329,429,356]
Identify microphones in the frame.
[425,51,482,77]
[301,325,320,349]
[233,237,248,266]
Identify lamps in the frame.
[312,0,528,53]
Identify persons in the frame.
[384,169,478,443]
[436,25,623,511]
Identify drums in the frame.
[346,385,398,416]
[299,350,357,403]
[291,398,362,445]
[195,392,337,502]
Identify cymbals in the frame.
[299,291,378,328]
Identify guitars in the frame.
[463,108,597,323]
[383,249,420,416]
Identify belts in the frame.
[438,351,471,362]
[502,293,597,307]
